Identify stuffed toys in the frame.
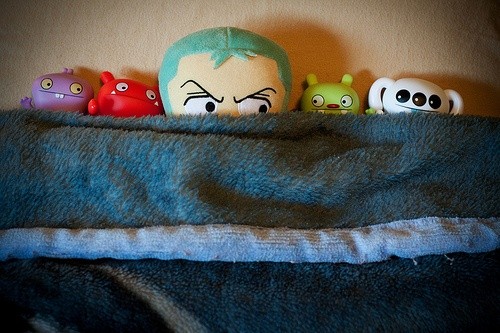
[157,28,292,115]
